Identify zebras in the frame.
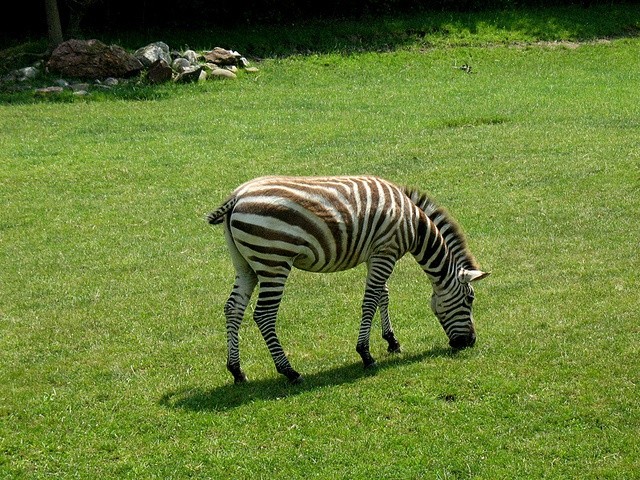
[207,173,492,385]
